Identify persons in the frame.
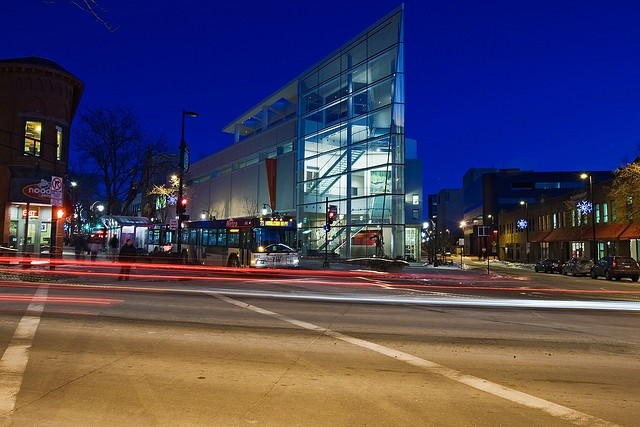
[70,235,87,260]
[118,239,137,280]
[108,233,120,261]
[89,233,101,261]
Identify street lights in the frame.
[580,172,598,264]
[176,110,199,254]
[520,199,531,264]
[487,214,496,260]
[87,200,104,234]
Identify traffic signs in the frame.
[50,176,64,208]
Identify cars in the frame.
[535,256,563,275]
[590,255,640,283]
[562,256,595,277]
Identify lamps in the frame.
[261,203,279,217]
[202,210,212,219]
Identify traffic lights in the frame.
[327,205,337,224]
[177,199,190,224]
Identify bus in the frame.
[147,212,303,269]
